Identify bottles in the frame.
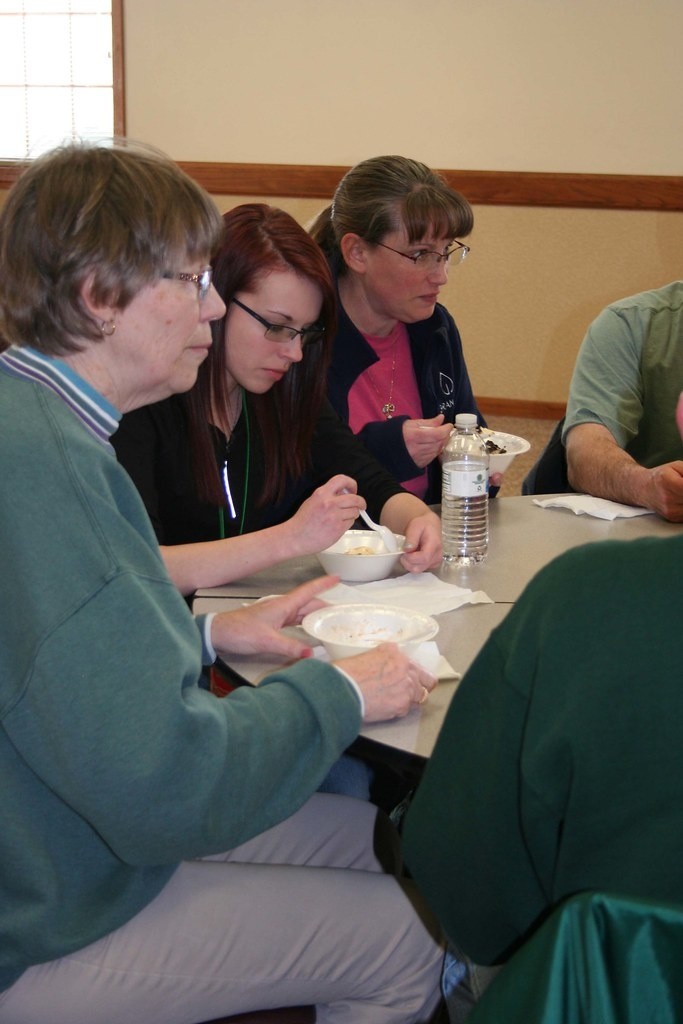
[440,413,490,568]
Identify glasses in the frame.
[228,295,326,352]
[160,267,212,300]
[379,239,472,273]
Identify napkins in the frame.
[529,494,658,521]
[244,570,496,629]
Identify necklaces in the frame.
[364,327,398,420]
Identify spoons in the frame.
[342,488,398,553]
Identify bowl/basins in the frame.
[301,604,439,658]
[482,430,531,476]
[315,528,409,582]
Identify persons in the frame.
[109,202,445,580]
[393,535,682,967]
[563,280,682,523]
[0,138,446,1024]
[304,155,503,501]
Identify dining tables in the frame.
[192,487,683,766]
